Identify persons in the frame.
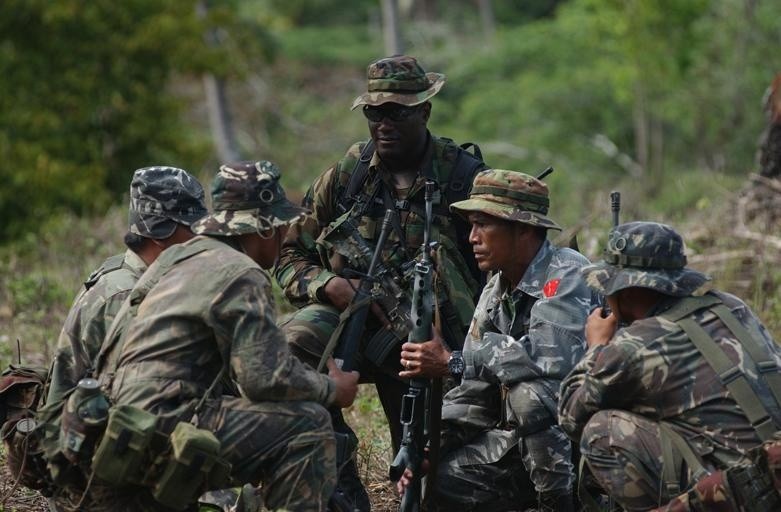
[398,168,607,510]
[92,158,364,512]
[276,53,500,511]
[42,165,209,512]
[554,218,781,509]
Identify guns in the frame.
[602,192,620,318]
[389,182,443,510]
[328,209,396,510]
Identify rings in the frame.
[405,360,410,368]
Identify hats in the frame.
[351,54,447,113]
[129,165,210,240]
[448,167,564,234]
[188,157,312,237]
[577,221,714,300]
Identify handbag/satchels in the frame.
[1,360,46,491]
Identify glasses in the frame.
[362,103,424,122]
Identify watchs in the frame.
[449,350,465,381]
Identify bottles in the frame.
[11,416,46,490]
[59,379,109,469]
